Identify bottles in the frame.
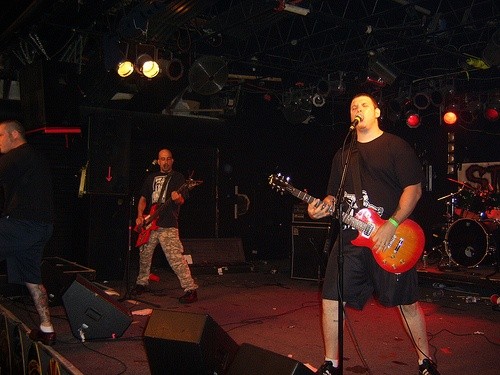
[433,283,446,289]
[423,251,429,266]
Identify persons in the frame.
[0,121,56,345]
[129,149,199,303]
[307,93,439,375]
[469,166,492,189]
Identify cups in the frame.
[490,293,500,304]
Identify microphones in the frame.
[349,114,363,131]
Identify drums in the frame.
[453,190,485,219]
[485,194,500,221]
[444,218,491,269]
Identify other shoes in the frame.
[179,290,198,303]
[130,285,148,294]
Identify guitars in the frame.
[267,172,425,275]
[133,177,204,248]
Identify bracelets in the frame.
[389,218,398,228]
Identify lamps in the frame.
[103,40,183,81]
[312,51,500,128]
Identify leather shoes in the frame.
[29,327,56,345]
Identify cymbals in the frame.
[447,177,476,191]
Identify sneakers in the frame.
[313,360,340,375]
[417,357,440,375]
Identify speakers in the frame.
[143,307,240,375]
[76,113,133,194]
[67,193,132,285]
[289,224,329,281]
[56,269,130,341]
[230,343,318,375]
